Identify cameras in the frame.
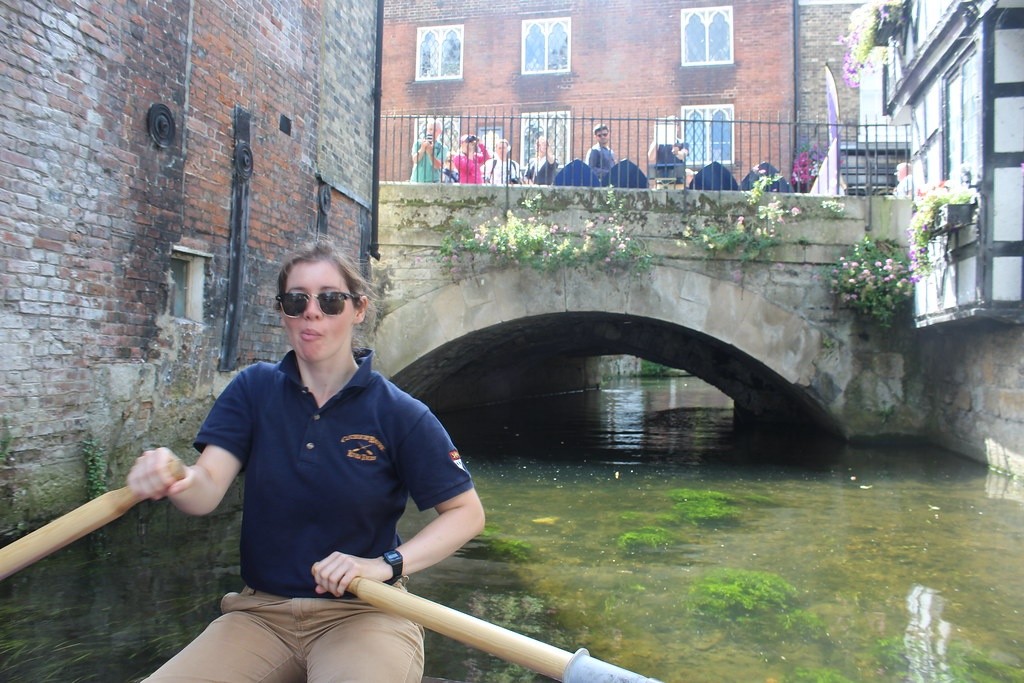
[426,134,433,143]
[466,136,476,142]
[510,177,522,184]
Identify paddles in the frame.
[311,562,660,683]
[0,459,186,579]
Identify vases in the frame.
[929,204,973,240]
[874,19,895,46]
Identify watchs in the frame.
[382,548,403,585]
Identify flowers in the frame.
[900,177,976,286]
[838,1,905,88]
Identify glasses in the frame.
[275,292,360,318]
[597,132,608,137]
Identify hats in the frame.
[461,134,473,143]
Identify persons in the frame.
[525,137,558,185]
[648,116,689,189]
[128,243,486,683]
[893,162,912,197]
[454,134,490,183]
[488,139,520,184]
[586,125,616,180]
[442,154,458,182]
[410,121,447,183]
